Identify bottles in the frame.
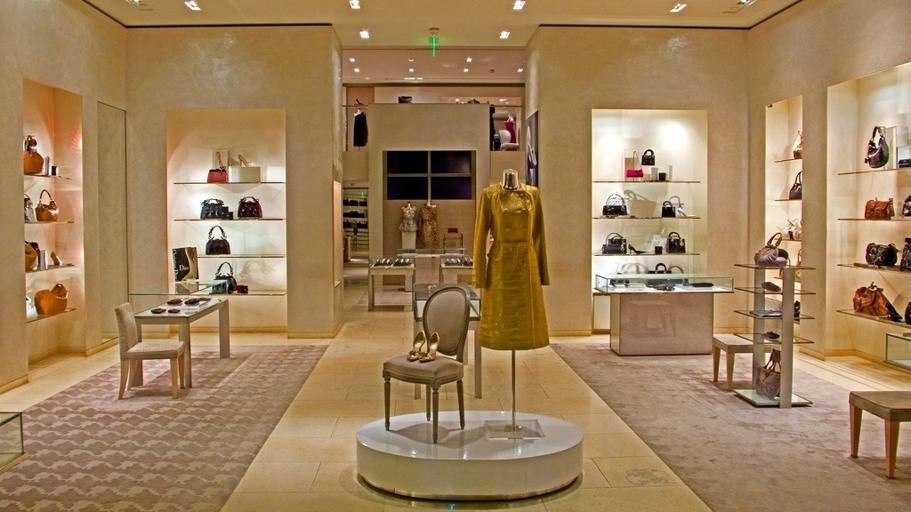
[655,246,662,254]
[45,156,59,176]
[39,250,49,270]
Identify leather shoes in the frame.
[445,255,473,266]
[375,255,410,266]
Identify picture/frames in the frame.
[526,111,538,187]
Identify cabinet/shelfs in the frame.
[0,412,29,478]
[439,261,474,289]
[592,179,702,333]
[596,274,733,357]
[367,263,416,312]
[343,187,369,258]
[171,181,286,298]
[23,173,77,324]
[775,158,804,324]
[734,263,816,410]
[836,166,911,379]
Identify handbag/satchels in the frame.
[212,261,238,294]
[853,192,911,325]
[792,130,803,160]
[602,189,685,217]
[23,132,68,320]
[200,198,223,219]
[752,348,782,400]
[754,232,783,267]
[207,151,228,183]
[205,225,231,255]
[237,196,262,218]
[609,261,714,292]
[778,170,804,325]
[864,125,890,168]
[624,148,666,181]
[600,231,627,255]
[666,231,686,253]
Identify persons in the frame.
[399,202,420,248]
[421,202,441,247]
[471,169,553,348]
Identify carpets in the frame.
[550,342,911,512]
[0,344,332,512]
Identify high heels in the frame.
[677,206,700,219]
[407,330,440,363]
[628,244,646,254]
[238,154,252,168]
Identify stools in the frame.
[847,391,911,477]
[712,334,798,391]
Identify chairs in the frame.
[381,286,471,443]
[115,301,186,399]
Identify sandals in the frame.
[354,99,368,107]
